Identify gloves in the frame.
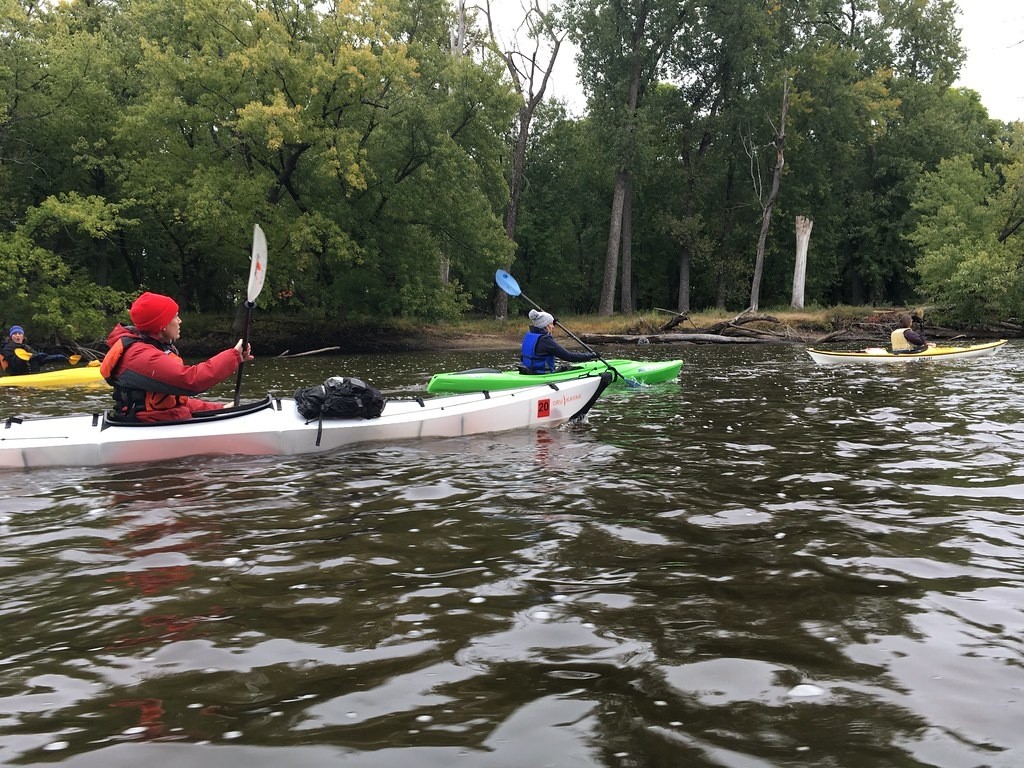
[551,315,558,325]
[588,351,601,359]
[37,352,48,360]
[56,354,67,365]
[921,336,925,341]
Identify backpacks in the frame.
[294,375,386,424]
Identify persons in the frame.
[521,309,601,375]
[3,326,65,376]
[891,314,927,351]
[100,292,254,422]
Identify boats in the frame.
[1,366,618,469]
[428,359,684,394]
[0,359,108,387]
[804,339,1008,366]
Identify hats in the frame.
[528,309,553,328]
[129,292,180,333]
[9,326,24,337]
[899,314,911,328]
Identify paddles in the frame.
[916,309,927,346]
[495,269,643,388]
[14,348,82,366]
[232,222,267,407]
